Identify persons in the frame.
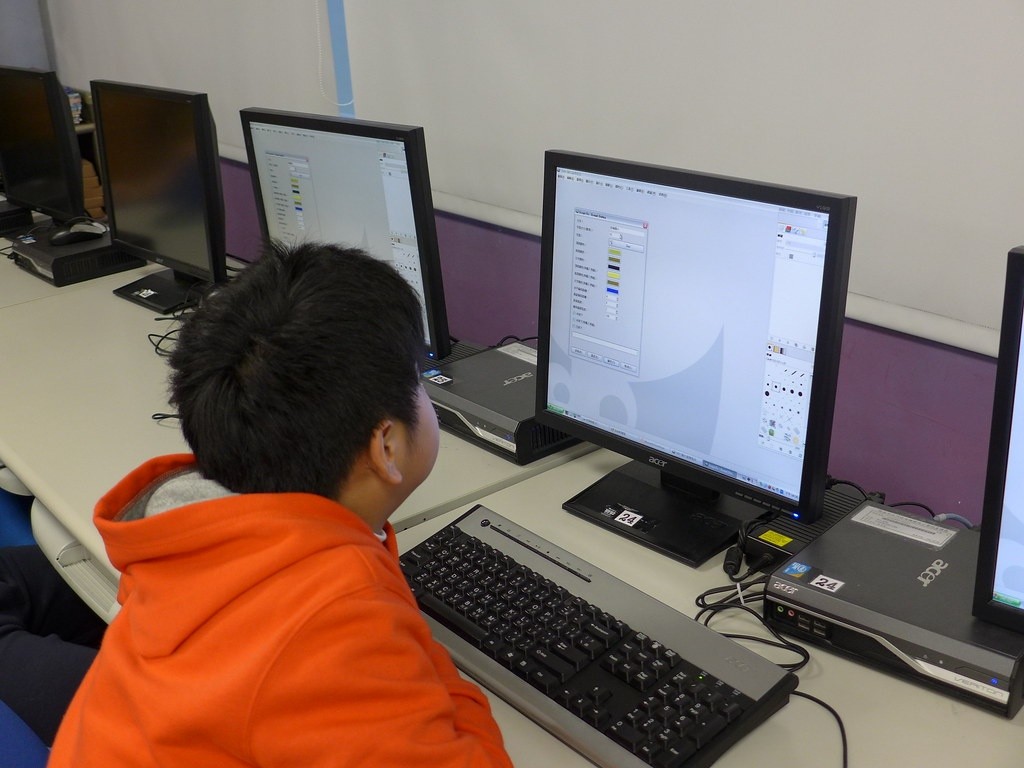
[46,235,514,768]
[0,545,109,748]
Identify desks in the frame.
[0,198,1024,768]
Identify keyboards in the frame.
[398,502,798,768]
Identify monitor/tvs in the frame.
[972,245,1024,637]
[239,107,453,360]
[89,79,231,314]
[0,65,92,228]
[533,151,858,568]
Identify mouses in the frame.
[48,221,103,246]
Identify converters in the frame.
[742,486,868,576]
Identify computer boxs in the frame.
[0,199,34,236]
[12,219,147,286]
[765,500,1024,719]
[420,341,591,466]
